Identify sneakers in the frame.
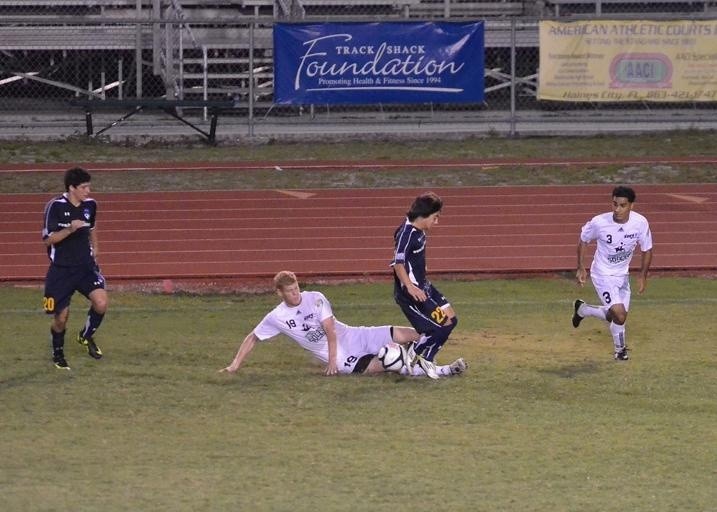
[572,299,584,327]
[77,332,102,359]
[615,351,628,360]
[406,341,467,379]
[52,357,70,369]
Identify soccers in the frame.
[379,344,407,371]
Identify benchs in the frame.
[69,97,235,146]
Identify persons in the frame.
[39,166,108,373]
[389,191,457,380]
[571,184,654,362]
[215,269,468,378]
